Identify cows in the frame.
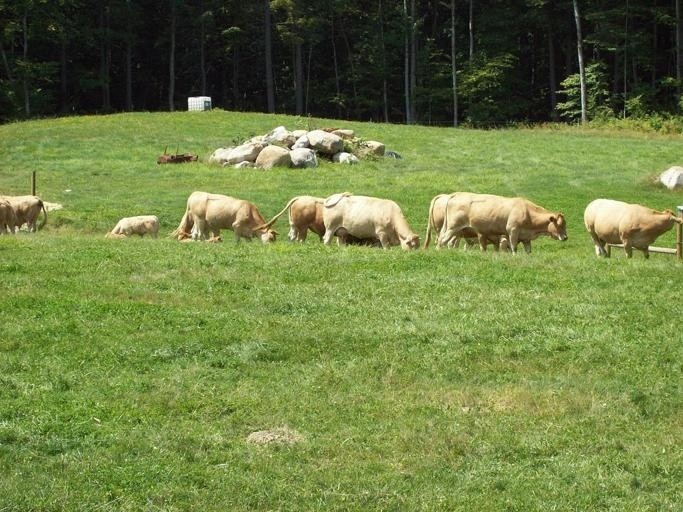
[0,195,47,235]
[165,191,281,244]
[423,191,569,256]
[584,198,683,259]
[110,215,159,239]
[251,191,422,252]
